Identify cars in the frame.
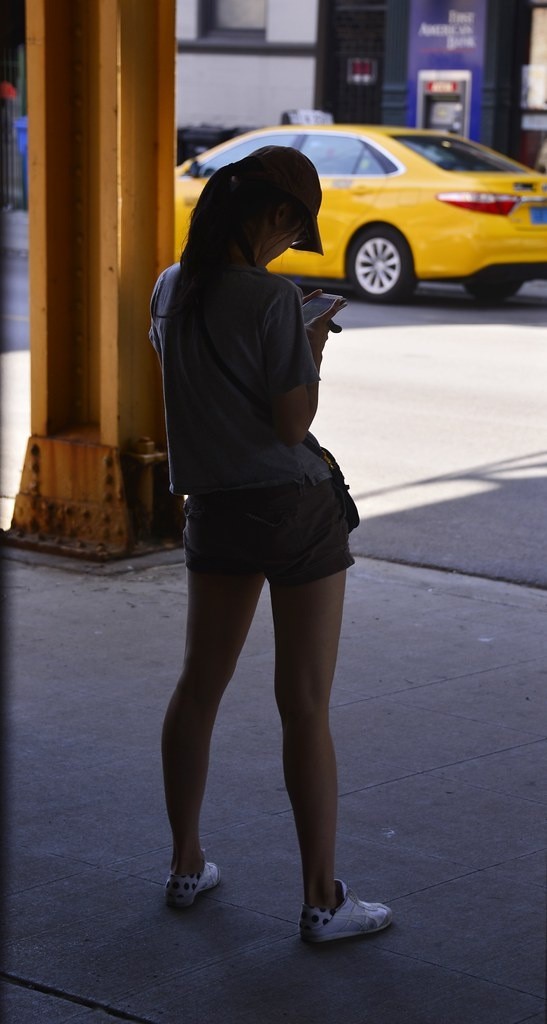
[176,125,547,303]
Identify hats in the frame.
[245,147,324,256]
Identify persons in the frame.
[145,146,391,943]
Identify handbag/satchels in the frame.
[322,447,360,534]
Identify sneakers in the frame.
[298,879,393,943]
[165,847,221,910]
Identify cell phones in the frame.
[302,292,343,325]
